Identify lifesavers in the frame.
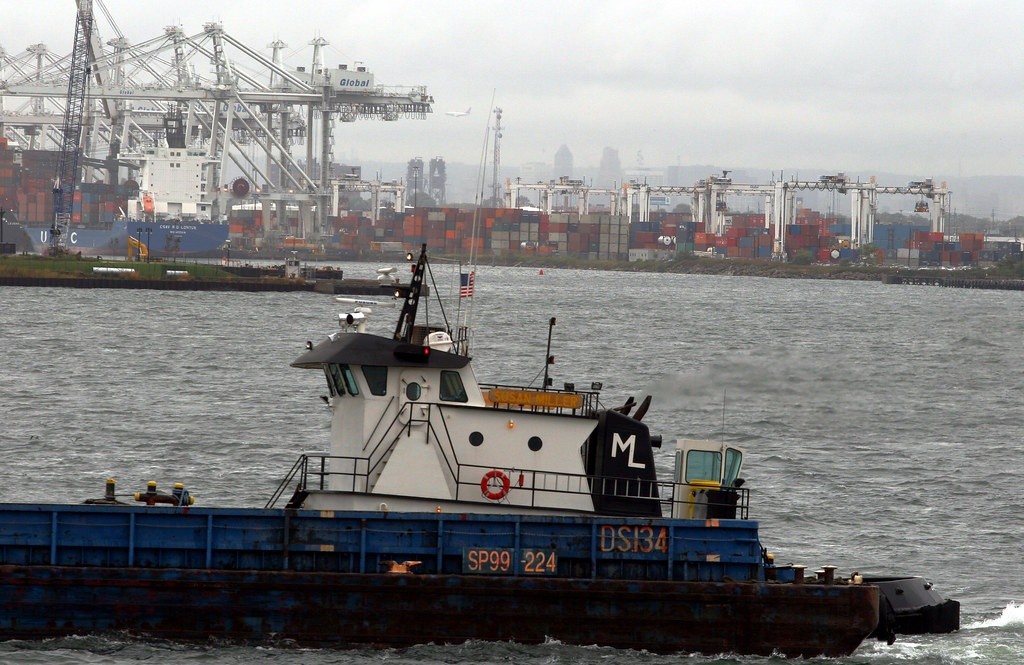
[480,470,512,500]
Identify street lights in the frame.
[137,228,152,263]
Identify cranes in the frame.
[0,0,435,238]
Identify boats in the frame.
[0,242,882,658]
